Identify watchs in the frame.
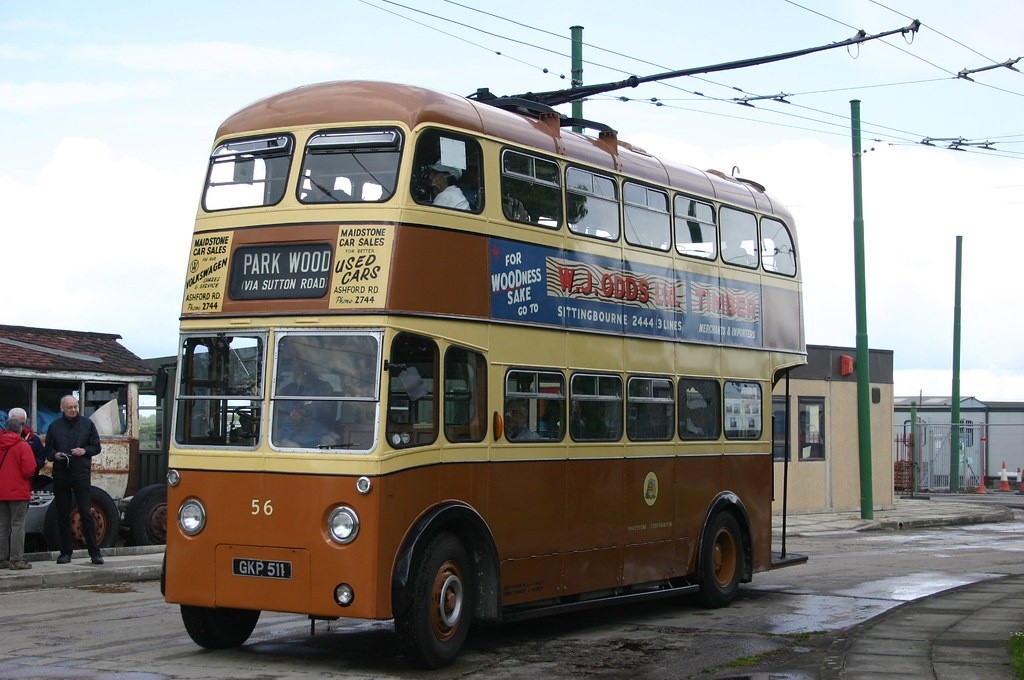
[303,409,309,416]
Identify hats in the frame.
[428,159,462,180]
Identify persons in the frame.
[45,394,104,564]
[428,158,472,212]
[301,164,354,202]
[0,407,46,570]
[504,398,541,440]
[538,399,560,438]
[255,358,338,447]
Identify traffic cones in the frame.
[1015,472,1024,494]
[994,461,1014,493]
[976,476,987,494]
[1014,467,1022,489]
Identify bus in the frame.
[153,79,809,671]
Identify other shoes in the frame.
[57,553,71,564]
[0,561,10,569]
[8,560,32,570]
[90,554,103,564]
